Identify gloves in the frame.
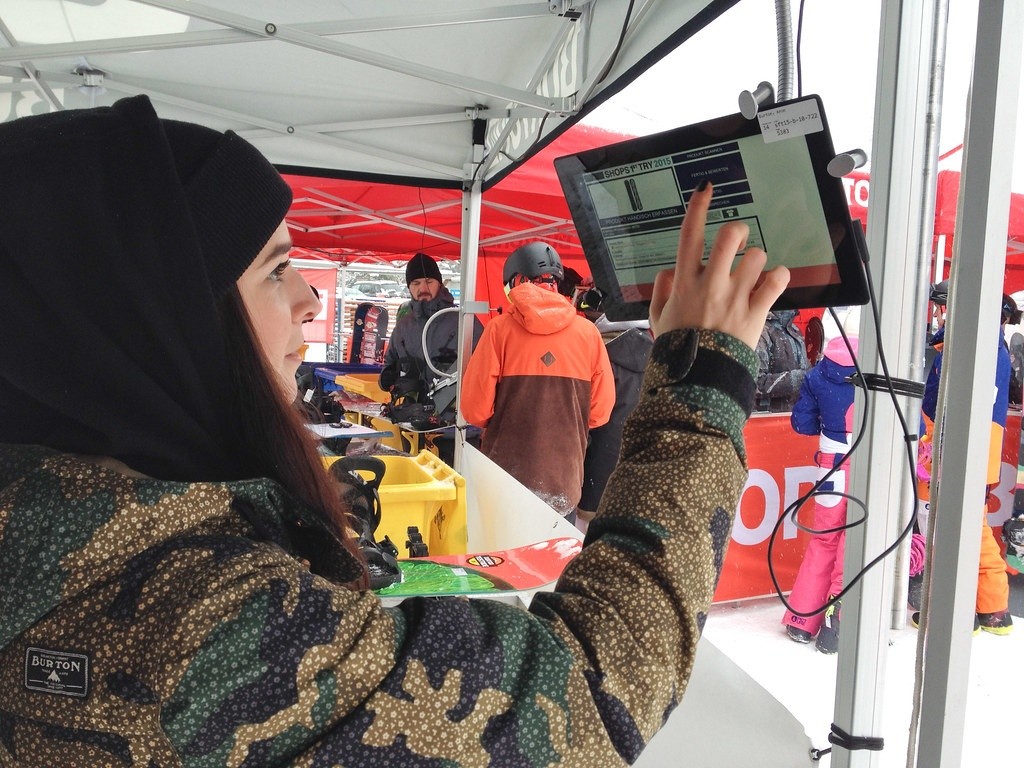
[381,366,397,390]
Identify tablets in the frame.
[552,94,869,322]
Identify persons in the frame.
[576,291,651,523]
[755,309,812,411]
[0,92,792,768]
[379,254,483,453]
[912,280,1014,636]
[783,306,862,653]
[461,241,615,526]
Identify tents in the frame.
[272,124,1024,471]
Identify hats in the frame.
[157,118,293,308]
[842,307,861,335]
[405,253,442,289]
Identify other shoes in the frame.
[977,611,1014,634]
[911,612,982,636]
[815,617,840,654]
[786,625,812,643]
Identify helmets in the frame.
[503,241,564,286]
[1001,295,1017,325]
[929,279,949,305]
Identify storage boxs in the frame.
[305,363,468,560]
